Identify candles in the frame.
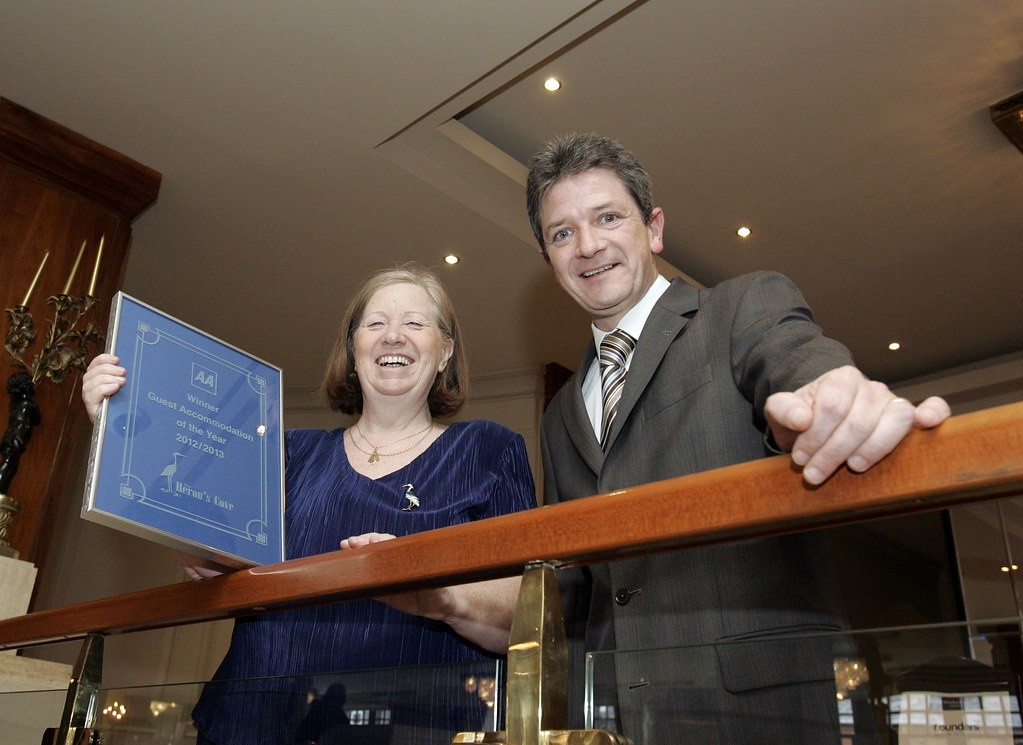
[59,238,88,297]
[87,233,106,297]
[19,248,51,314]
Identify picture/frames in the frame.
[78,289,288,571]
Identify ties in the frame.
[599,329,638,452]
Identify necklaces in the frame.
[348,420,434,466]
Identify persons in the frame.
[77,267,541,745]
[337,123,955,745]
